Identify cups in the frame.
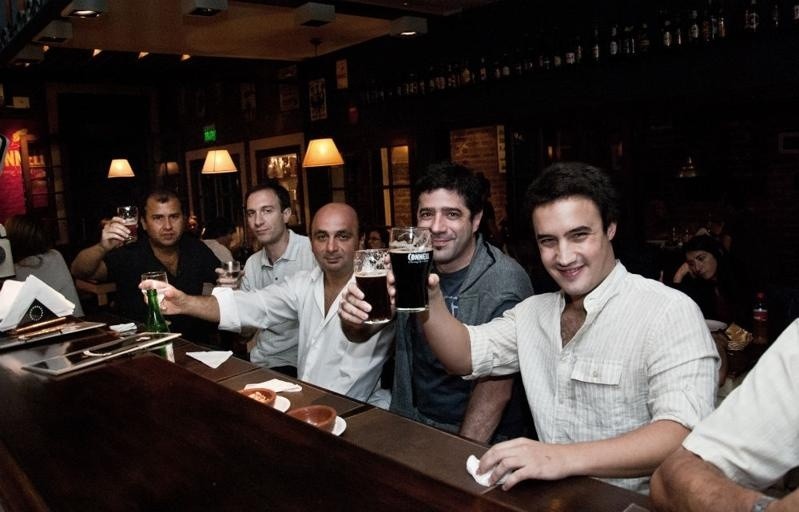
[116,205,139,245]
[351,247,393,325]
[389,226,435,311]
[221,257,241,291]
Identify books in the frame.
[0,275,76,329]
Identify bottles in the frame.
[146,288,175,362]
[754,292,770,344]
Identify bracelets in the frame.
[746,492,777,512]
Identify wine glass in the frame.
[139,270,173,327]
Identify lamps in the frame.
[9,0,113,67]
[106,158,137,180]
[180,0,228,20]
[300,135,345,169]
[293,2,337,30]
[388,16,429,40]
[201,149,239,219]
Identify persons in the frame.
[199,217,237,266]
[649,315,798,511]
[0,208,86,324]
[394,164,719,491]
[338,164,535,445]
[138,199,398,410]
[66,187,223,343]
[661,231,756,331]
[211,183,322,369]
[187,215,199,233]
[359,223,393,255]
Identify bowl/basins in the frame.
[285,404,336,433]
[239,387,277,408]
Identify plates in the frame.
[273,394,290,413]
[703,319,726,336]
[334,415,348,436]
